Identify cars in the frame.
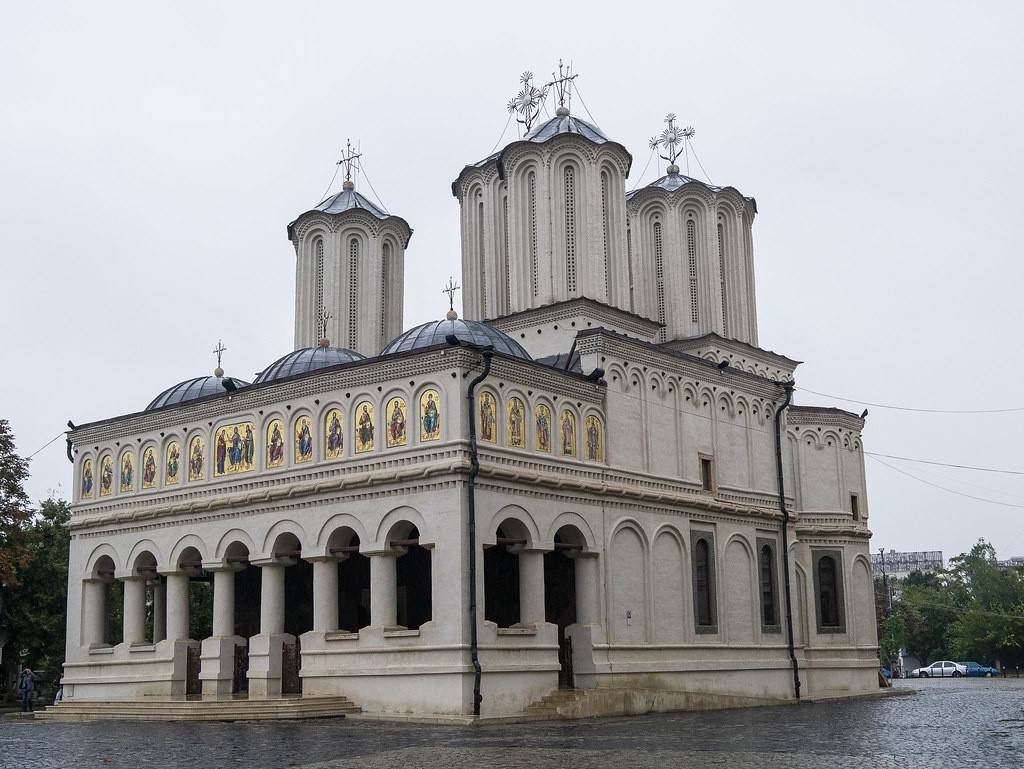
[956,662,998,677]
[882,668,893,677]
[911,660,969,678]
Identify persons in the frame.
[19,668,37,712]
[83,461,94,497]
[481,394,599,463]
[121,454,133,491]
[167,445,179,483]
[144,449,155,486]
[216,392,441,477]
[102,457,113,494]
[54,671,64,706]
[190,438,205,480]
